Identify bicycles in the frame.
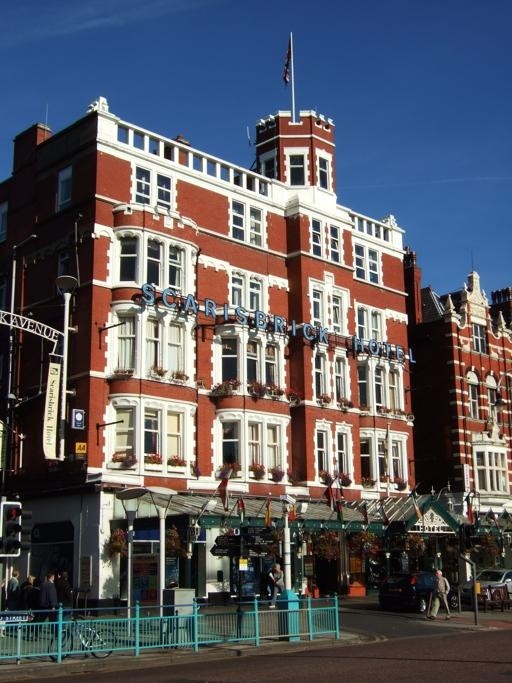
[48,613,117,658]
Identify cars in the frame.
[463,569,512,604]
[378,571,459,612]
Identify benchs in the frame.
[477,584,512,613]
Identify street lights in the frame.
[118,488,177,645]
[7,235,36,424]
[56,276,77,462]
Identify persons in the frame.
[268,562,285,609]
[1,569,71,640]
[428,569,451,618]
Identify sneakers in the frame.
[268,605,276,609]
[445,615,450,620]
[427,616,436,620]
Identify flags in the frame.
[484,510,499,532]
[336,500,343,523]
[464,495,473,523]
[501,510,512,523]
[324,477,345,512]
[290,504,296,522]
[377,505,390,526]
[264,497,272,528]
[236,497,246,525]
[409,489,423,522]
[360,504,369,524]
[218,467,233,510]
[282,36,291,85]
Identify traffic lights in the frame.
[3,505,22,554]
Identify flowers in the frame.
[104,528,128,560]
[211,377,407,417]
[112,451,406,491]
[317,529,425,562]
